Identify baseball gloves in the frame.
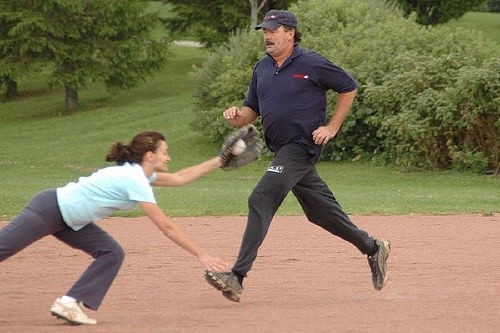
[219,124,263,173]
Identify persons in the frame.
[0,125,262,326]
[205,11,392,303]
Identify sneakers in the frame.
[368,239,391,290]
[204,269,242,302]
[50,298,97,325]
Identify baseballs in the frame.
[232,138,246,156]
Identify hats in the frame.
[255,9,297,30]
